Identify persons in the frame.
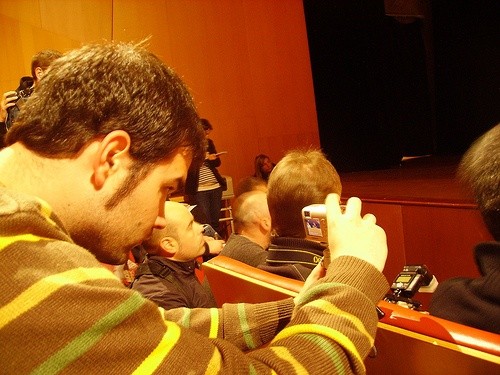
[0,39,388,375]
[429,121,500,333]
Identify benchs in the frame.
[201,256,500,375]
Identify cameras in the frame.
[382,264,439,310]
[302,204,346,242]
